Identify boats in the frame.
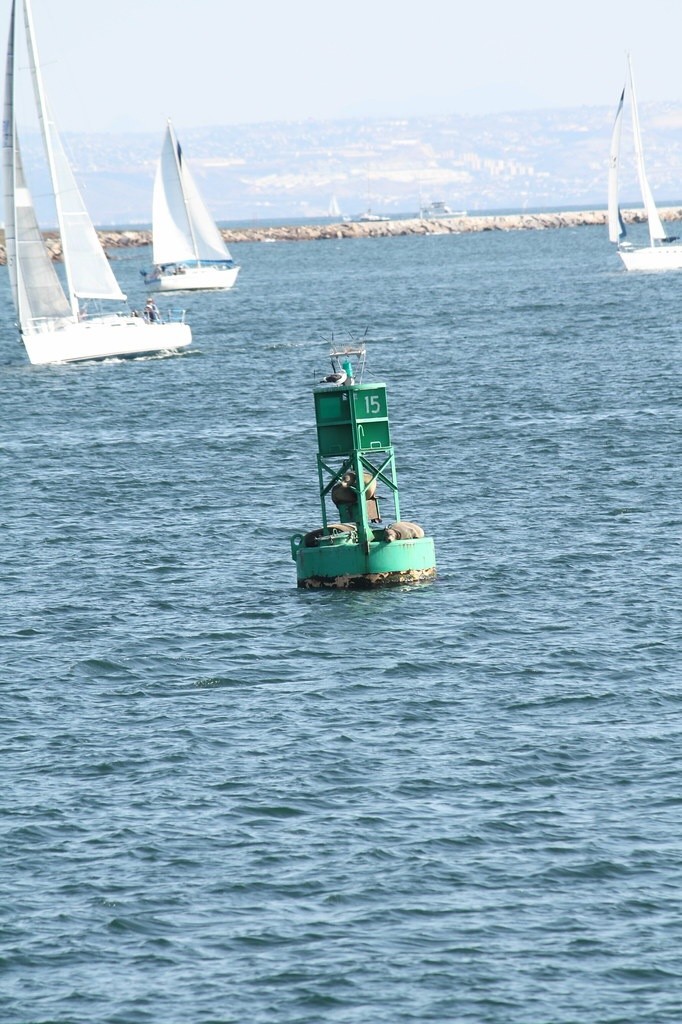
[291,353,436,579]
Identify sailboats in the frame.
[602,51,682,271]
[147,122,239,290]
[2,0,194,366]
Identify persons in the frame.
[144,297,160,322]
